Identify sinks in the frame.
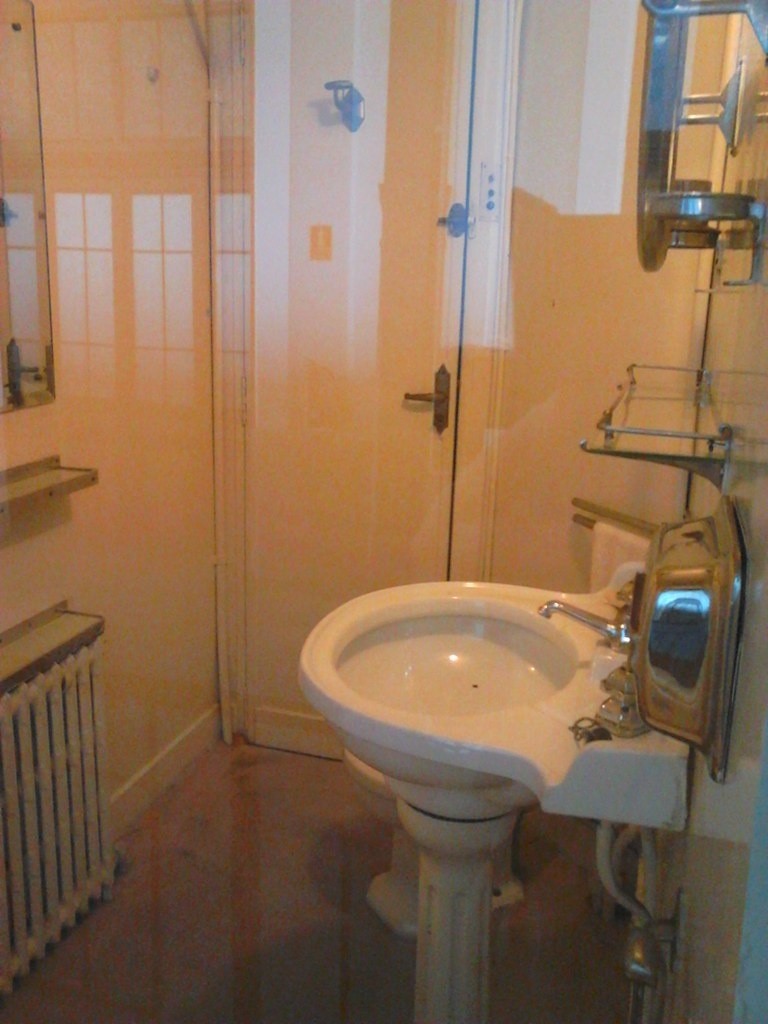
[297,562,688,833]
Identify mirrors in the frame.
[0,0,57,417]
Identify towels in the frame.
[591,521,650,596]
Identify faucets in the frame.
[536,600,631,652]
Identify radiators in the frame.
[0,631,122,998]
